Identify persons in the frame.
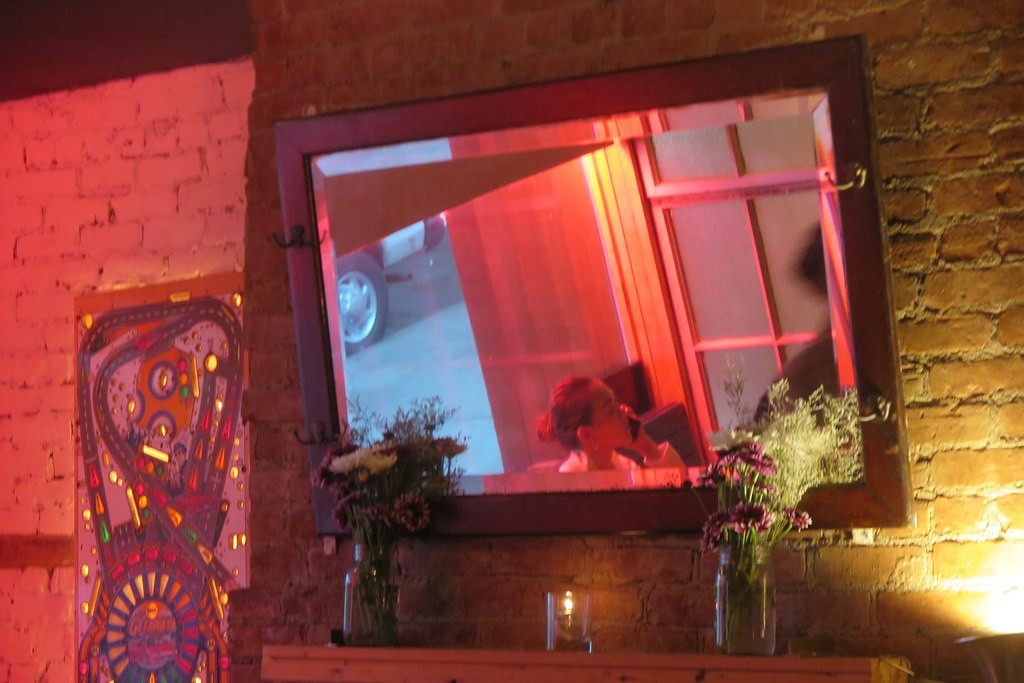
[753,224,837,422]
[536,375,687,474]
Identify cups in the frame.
[545,591,591,651]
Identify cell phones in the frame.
[628,417,641,442]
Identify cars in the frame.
[336,211,445,357]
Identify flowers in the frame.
[703,417,828,548]
[758,384,863,484]
[312,404,439,543]
[404,401,466,501]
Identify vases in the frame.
[344,542,402,646]
[718,546,778,655]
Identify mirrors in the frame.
[270,29,915,536]
